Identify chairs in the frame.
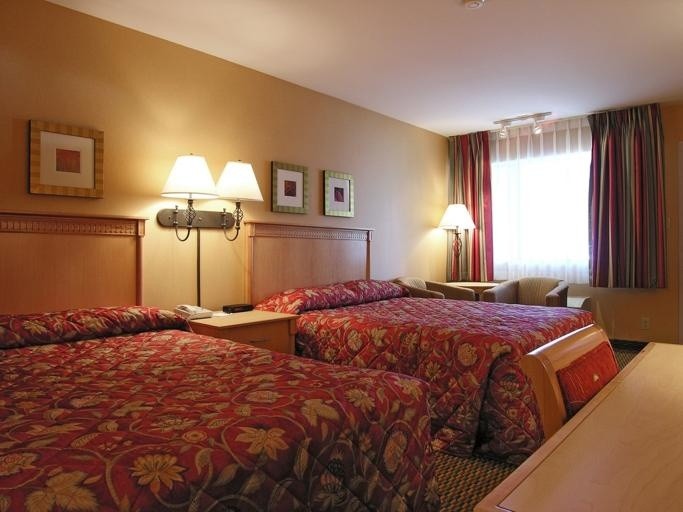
[480,276,569,306]
[519,323,621,438]
[394,277,475,301]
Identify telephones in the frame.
[173,304,213,319]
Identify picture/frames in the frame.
[29,120,104,198]
[324,169,355,218]
[271,161,309,216]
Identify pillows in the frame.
[0,305,184,348]
[258,280,407,314]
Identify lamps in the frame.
[497,120,512,139]
[532,116,544,135]
[161,153,216,242]
[442,204,475,281]
[216,161,264,240]
[464,0,484,13]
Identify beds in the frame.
[0,213,433,511]
[246,222,593,459]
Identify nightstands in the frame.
[189,309,301,355]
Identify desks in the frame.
[442,282,495,294]
[474,342,682,512]
